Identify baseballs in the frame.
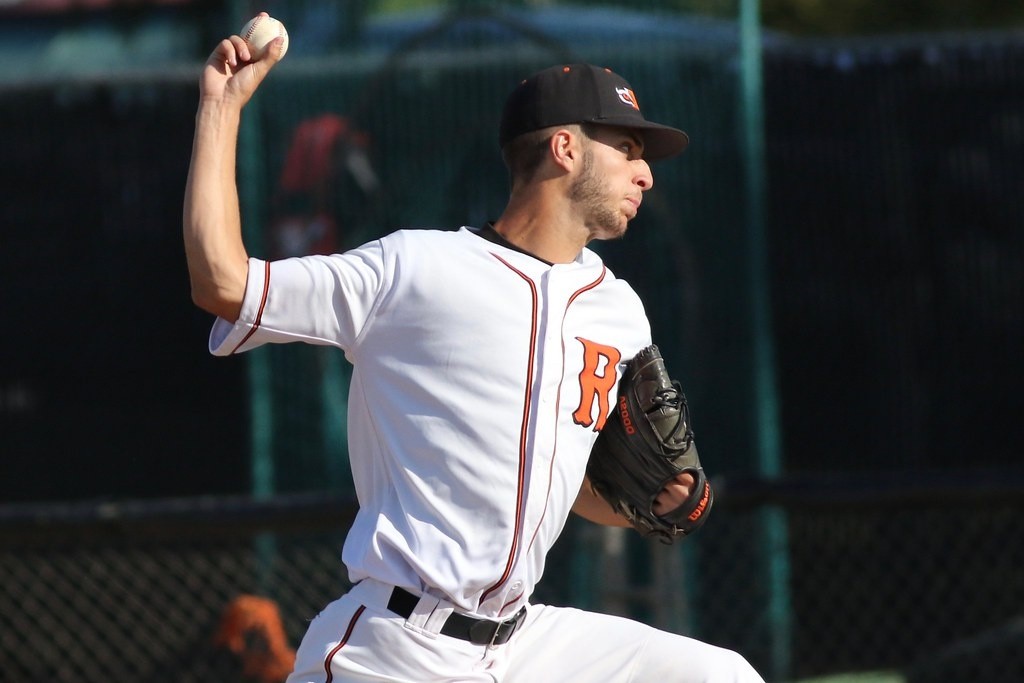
[241,13,290,60]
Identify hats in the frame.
[497,62,689,159]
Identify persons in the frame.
[182,12,766,683]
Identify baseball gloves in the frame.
[585,344,715,546]
